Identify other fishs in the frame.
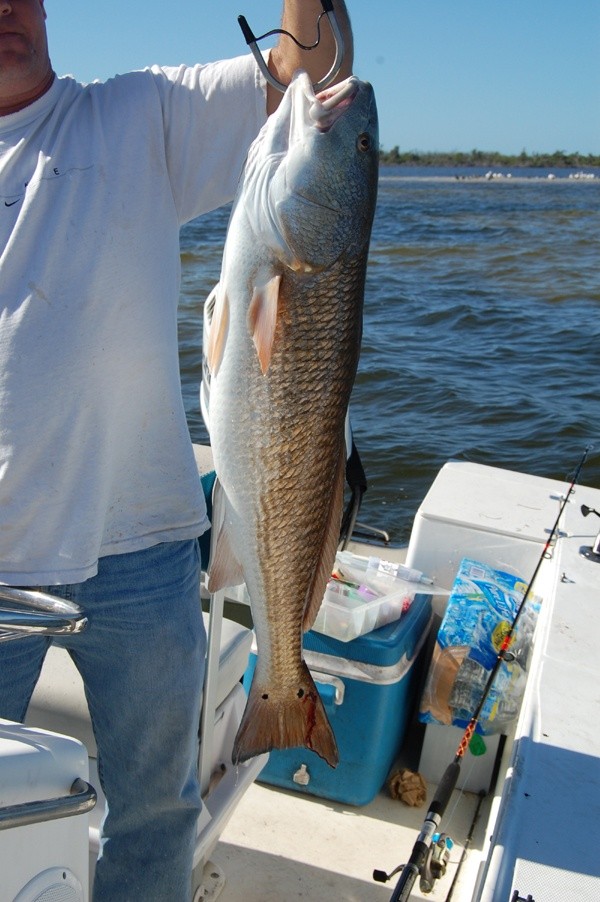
[198,69,381,769]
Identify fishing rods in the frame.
[371,440,592,902]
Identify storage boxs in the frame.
[244,594,436,806]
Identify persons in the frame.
[0,0,355,902]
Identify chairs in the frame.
[22,444,270,902]
